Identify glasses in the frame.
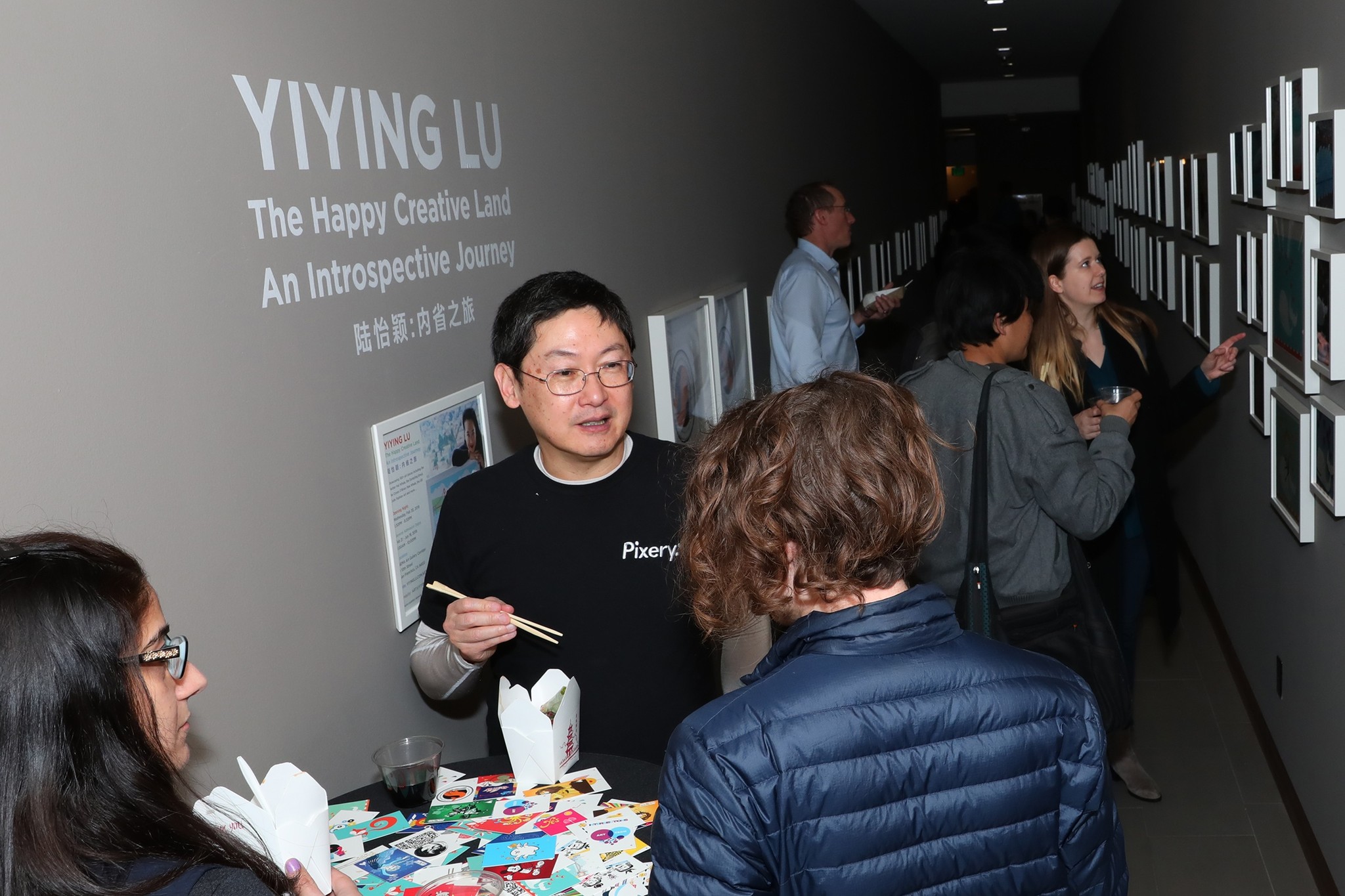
[814,200,854,215]
[510,356,643,397]
[112,629,191,684]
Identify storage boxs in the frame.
[192,760,333,896]
[495,669,580,785]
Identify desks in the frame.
[325,752,661,896]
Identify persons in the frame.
[1013,223,1246,801]
[883,246,1142,685]
[1,531,358,896]
[452,408,485,471]
[643,370,1133,895]
[408,269,773,768]
[767,181,904,394]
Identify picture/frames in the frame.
[1065,57,1344,544]
[699,280,757,423]
[766,207,950,351]
[370,380,496,633]
[647,296,718,449]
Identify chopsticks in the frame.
[425,580,562,646]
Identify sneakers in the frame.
[1102,741,1165,808]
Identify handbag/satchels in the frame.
[958,560,1143,750]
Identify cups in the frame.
[371,734,444,809]
[1095,384,1134,405]
